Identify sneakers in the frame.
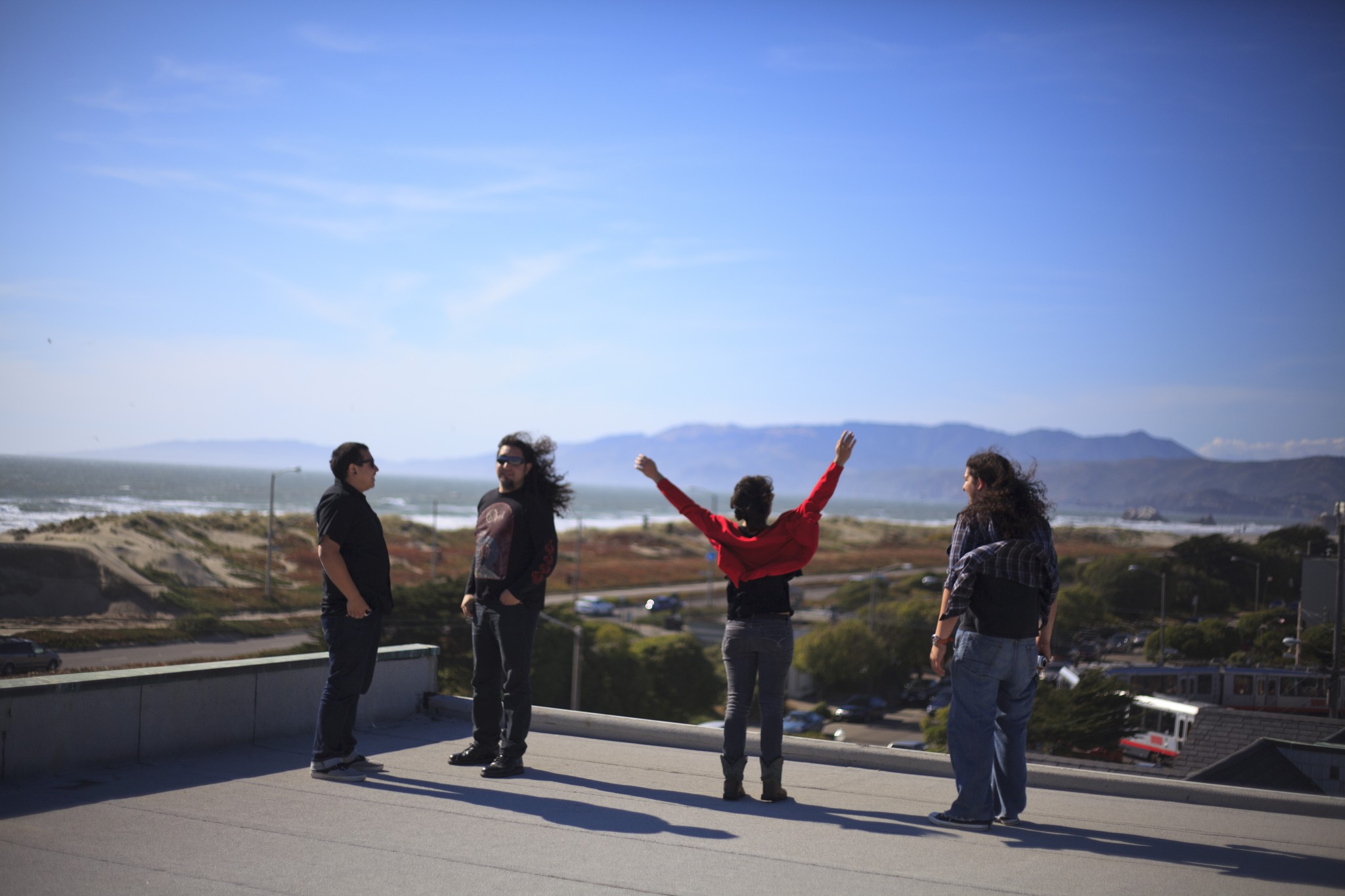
[998,815,1019,827]
[310,762,367,782]
[346,755,384,771]
[928,812,990,832]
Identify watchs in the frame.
[930,634,955,645]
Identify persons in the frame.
[929,450,1060,830]
[447,429,575,778]
[633,430,857,801]
[310,442,391,781]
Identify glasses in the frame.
[497,455,525,467]
[360,458,374,468]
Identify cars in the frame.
[0,637,63,680]
[1039,662,1059,684]
[834,692,889,724]
[782,710,824,738]
[889,740,930,752]
[652,594,683,613]
[575,595,616,618]
[1071,630,1152,660]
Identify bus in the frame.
[1113,692,1200,768]
[1055,663,1345,718]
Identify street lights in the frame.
[1231,555,1261,613]
[1284,622,1336,719]
[1128,563,1166,668]
[264,465,301,597]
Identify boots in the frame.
[720,754,749,799]
[758,755,788,800]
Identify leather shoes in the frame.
[448,742,499,766]
[480,753,525,779]
